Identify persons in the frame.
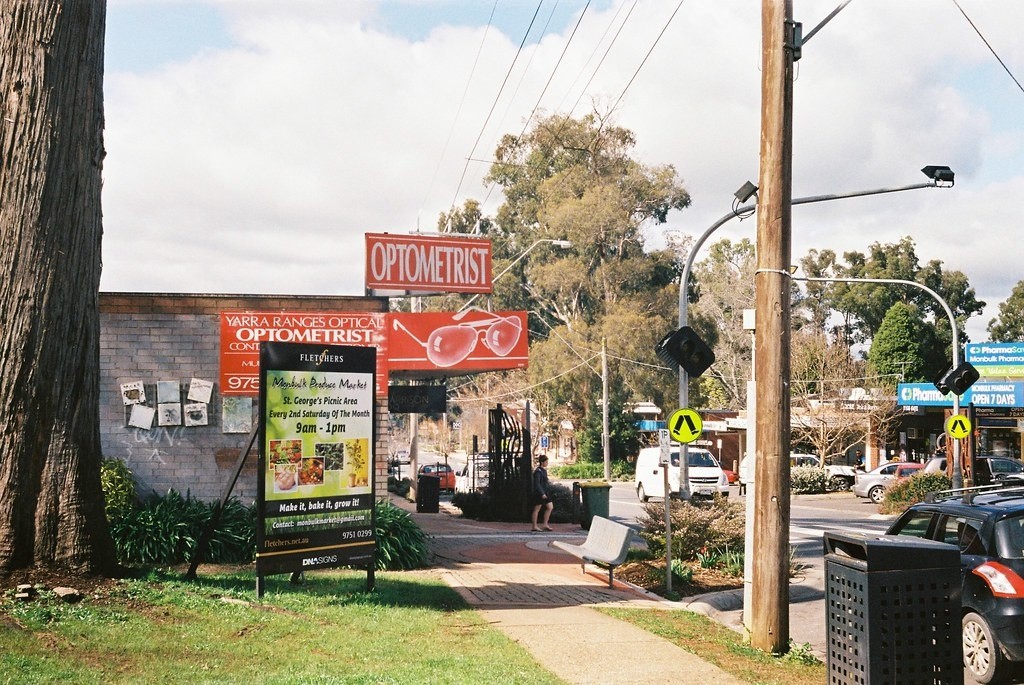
[531,454,554,531]
[572,446,576,461]
[854,450,866,474]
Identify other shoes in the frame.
[531,528,542,532]
[543,527,553,531]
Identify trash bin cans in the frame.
[577,481,612,531]
[416,474,440,513]
[823,529,965,685]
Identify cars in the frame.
[473,453,491,479]
[924,458,948,477]
[393,449,411,465]
[719,463,736,484]
[740,454,856,493]
[419,463,456,494]
[854,462,920,505]
[884,492,1024,685]
[974,457,1024,492]
[895,464,924,485]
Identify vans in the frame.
[635,447,728,503]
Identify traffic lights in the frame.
[945,363,980,396]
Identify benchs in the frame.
[552,515,635,589]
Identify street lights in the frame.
[456,239,575,313]
